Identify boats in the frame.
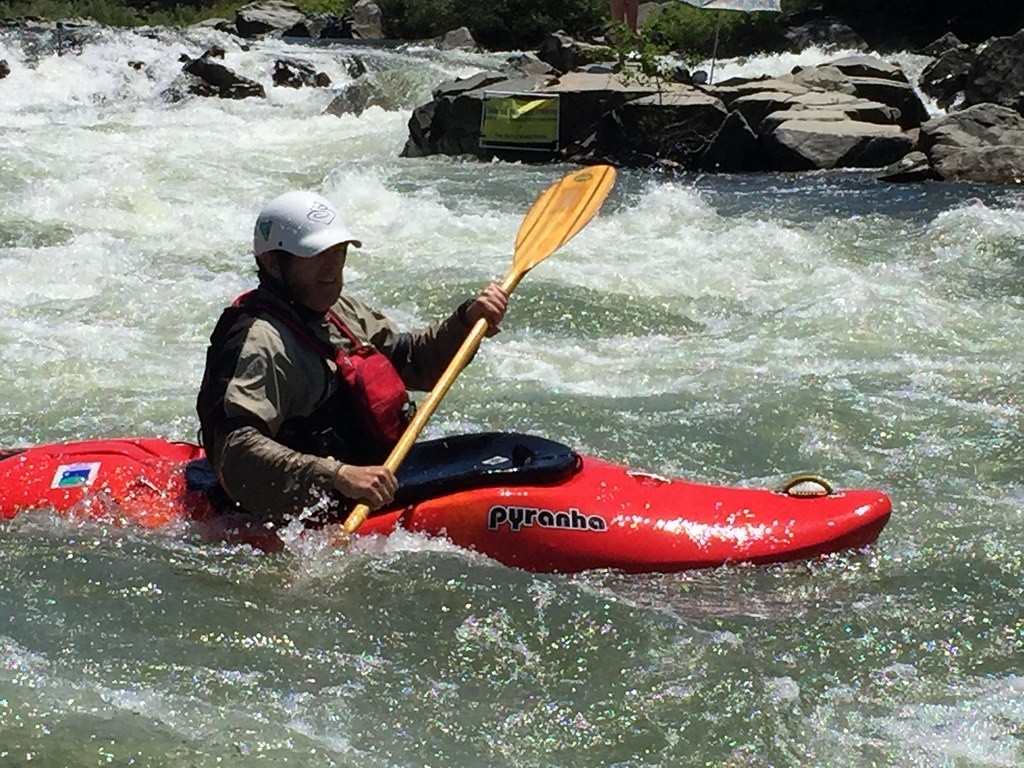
[0,429,892,573]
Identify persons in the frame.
[194,189,510,533]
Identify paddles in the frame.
[345,165,618,529]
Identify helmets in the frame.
[253,190,363,258]
